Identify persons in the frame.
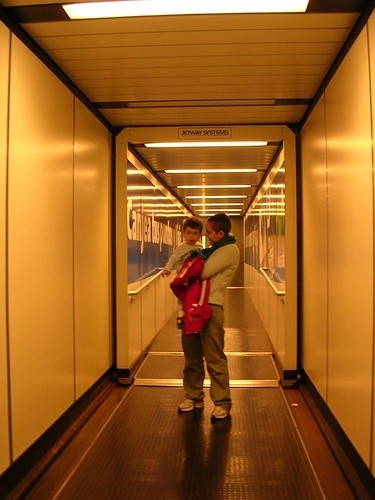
[161,218,204,329]
[179,214,239,418]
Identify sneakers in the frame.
[212,405,231,418]
[178,400,204,411]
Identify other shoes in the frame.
[176,316,184,329]
